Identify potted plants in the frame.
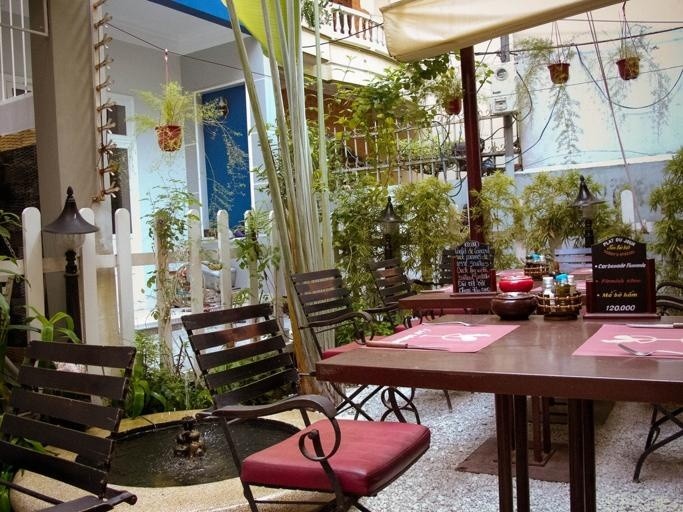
[520,35,584,166]
[428,70,463,115]
[129,82,224,152]
[585,24,671,135]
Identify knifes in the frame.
[365,341,450,352]
[625,322,682,329]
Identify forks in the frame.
[616,341,682,358]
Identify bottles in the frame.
[542,274,576,302]
[525,254,546,263]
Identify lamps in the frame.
[43,187,100,344]
[569,175,608,247]
[373,196,403,303]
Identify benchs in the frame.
[0,340,137,512]
[553,248,593,274]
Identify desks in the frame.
[399,269,587,314]
[320,312,683,512]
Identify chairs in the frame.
[634,400,683,482]
[438,249,454,287]
[367,257,469,333]
[655,281,683,316]
[291,268,422,424]
[181,303,429,512]
